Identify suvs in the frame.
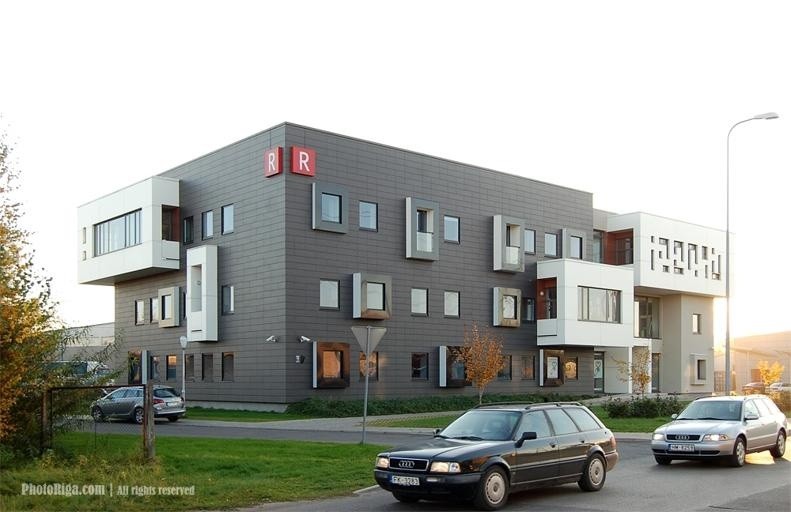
[89,384,188,427]
[372,395,624,512]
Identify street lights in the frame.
[178,334,188,419]
[722,110,780,395]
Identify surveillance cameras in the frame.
[299,335,312,343]
[264,335,276,344]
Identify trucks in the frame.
[37,360,115,390]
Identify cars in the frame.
[648,393,790,472]
[740,379,791,396]
